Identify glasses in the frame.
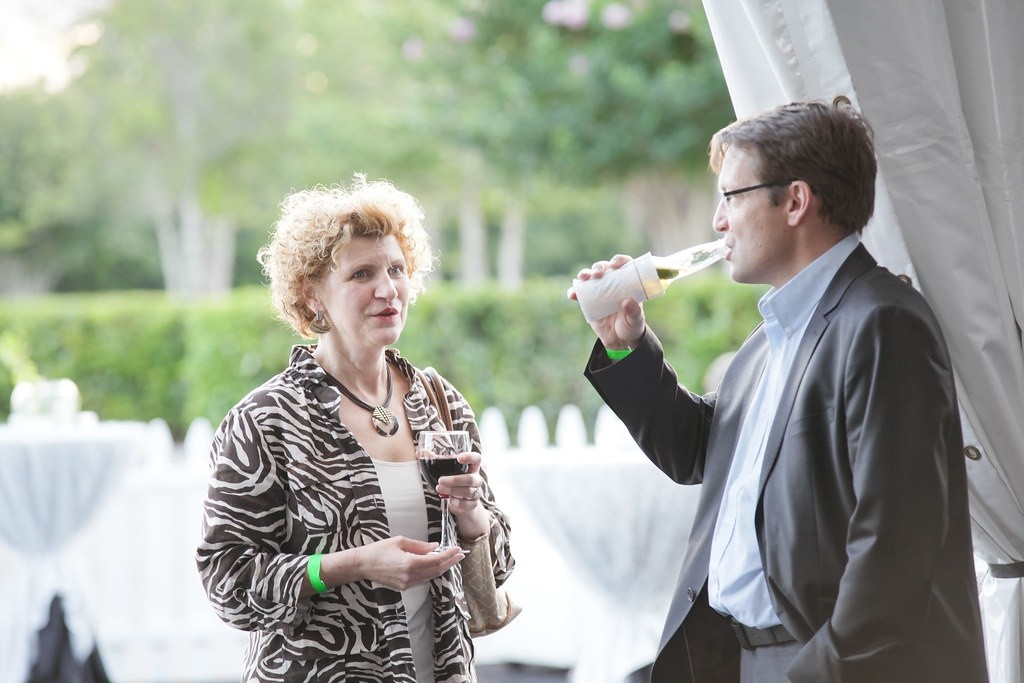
[720,179,816,208]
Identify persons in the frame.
[194,172,518,683]
[564,99,991,682]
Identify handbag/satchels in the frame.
[456,531,524,639]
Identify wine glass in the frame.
[418,431,471,555]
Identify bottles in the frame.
[572,237,730,323]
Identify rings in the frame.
[465,486,480,499]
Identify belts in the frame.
[731,619,797,651]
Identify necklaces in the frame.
[307,350,400,438]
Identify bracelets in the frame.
[306,548,335,599]
[460,520,492,542]
